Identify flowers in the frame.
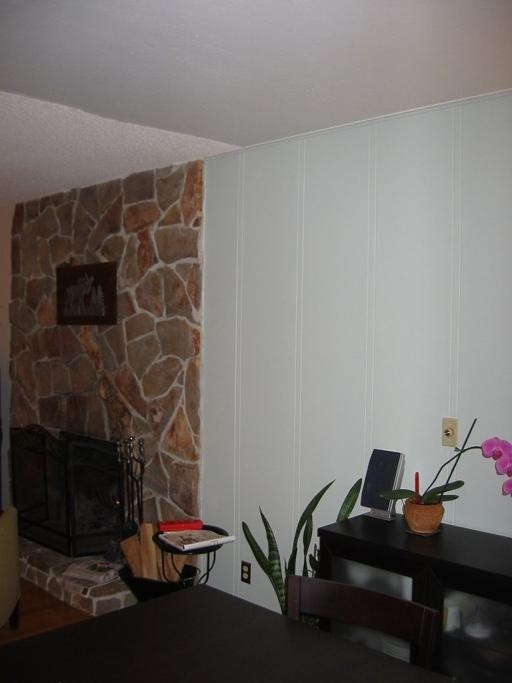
[373,435,510,499]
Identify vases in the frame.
[401,501,447,535]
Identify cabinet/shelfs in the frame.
[318,539,431,660]
[435,570,512,681]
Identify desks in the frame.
[152,525,226,582]
[1,584,446,681]
[318,504,510,603]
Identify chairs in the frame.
[287,576,445,671]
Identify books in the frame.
[64,558,123,583]
[158,528,235,551]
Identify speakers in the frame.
[360,448,404,521]
